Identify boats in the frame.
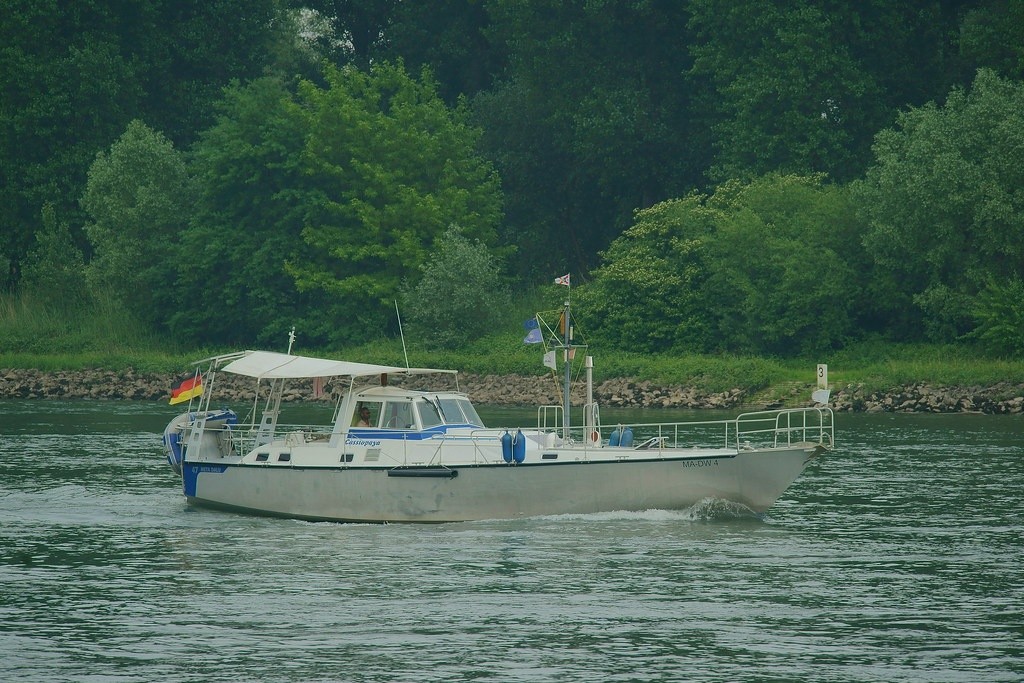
[177,271,837,525]
[161,408,237,475]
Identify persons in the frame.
[356,407,374,427]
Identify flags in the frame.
[555,274,569,285]
[543,351,557,370]
[169,368,202,405]
[523,328,543,344]
[568,349,576,360]
